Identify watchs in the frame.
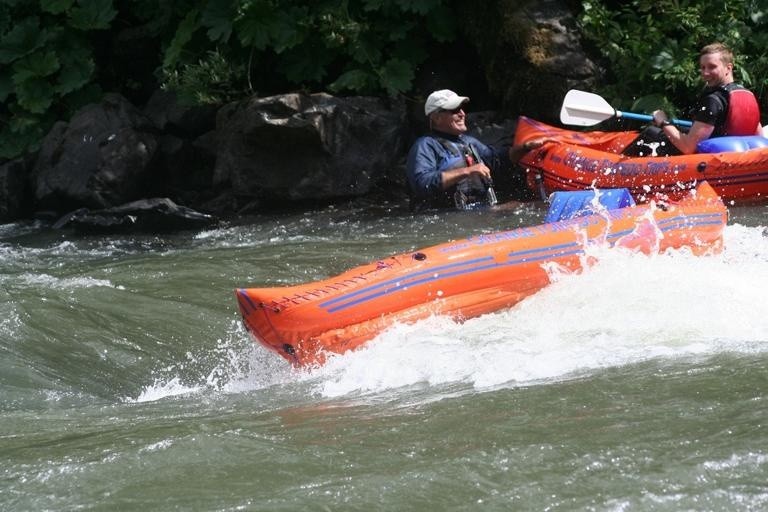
[660,119,673,130]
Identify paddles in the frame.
[560,90,693,127]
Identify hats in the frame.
[424,87,472,114]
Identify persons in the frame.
[618,42,762,157]
[404,88,496,211]
